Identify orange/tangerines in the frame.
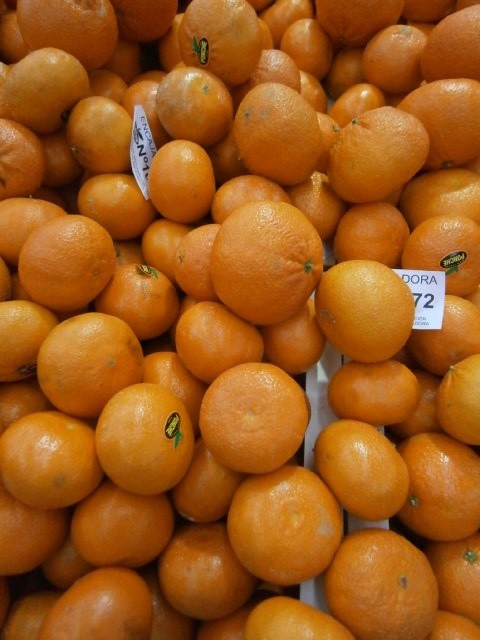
[0,0,479,640]
